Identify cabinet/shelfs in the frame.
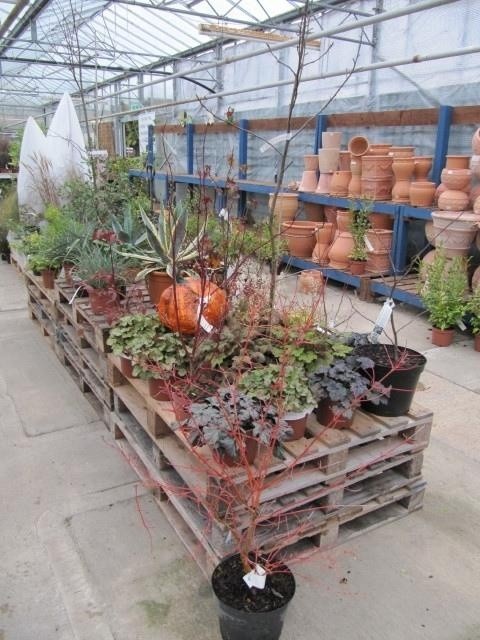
[124,108,479,327]
[14,257,435,590]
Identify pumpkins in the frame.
[160,277,229,333]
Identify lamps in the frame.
[198,24,320,50]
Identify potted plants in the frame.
[2,136,478,466]
